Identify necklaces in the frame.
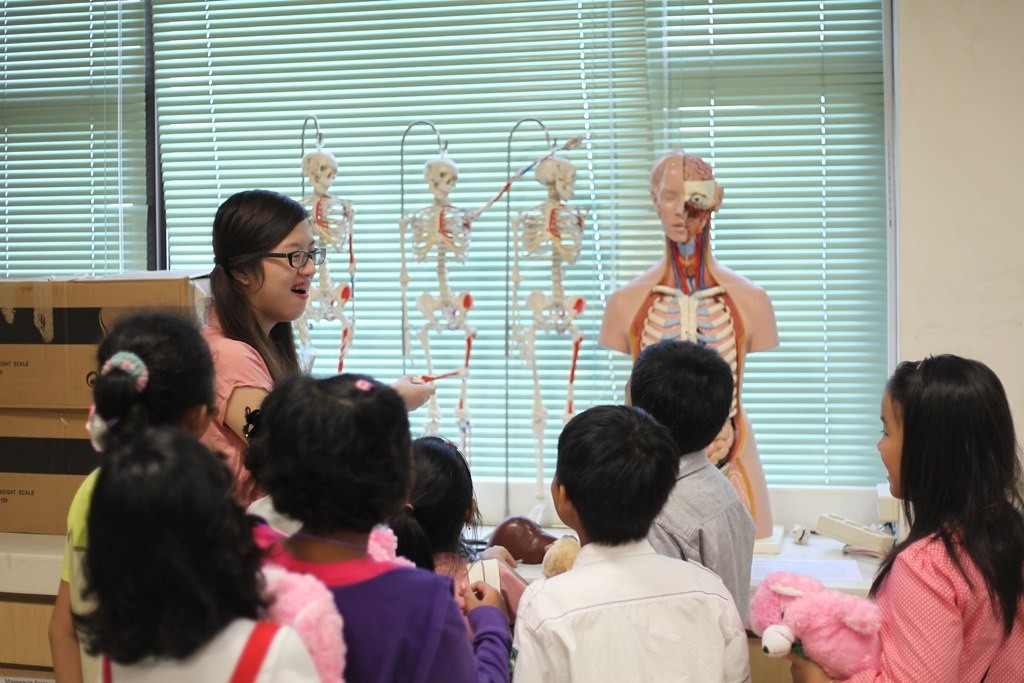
[295,532,368,548]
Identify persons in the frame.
[199,190,436,511]
[509,404,753,682]
[408,436,530,646]
[48,313,215,683]
[243,372,515,683]
[787,355,1024,683]
[67,431,320,683]
[599,151,779,540]
[625,339,756,628]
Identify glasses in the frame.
[261,248,326,268]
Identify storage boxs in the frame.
[0,277,196,410]
[0,408,103,537]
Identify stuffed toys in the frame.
[749,573,882,679]
[253,566,346,682]
[367,524,416,568]
[542,537,580,578]
